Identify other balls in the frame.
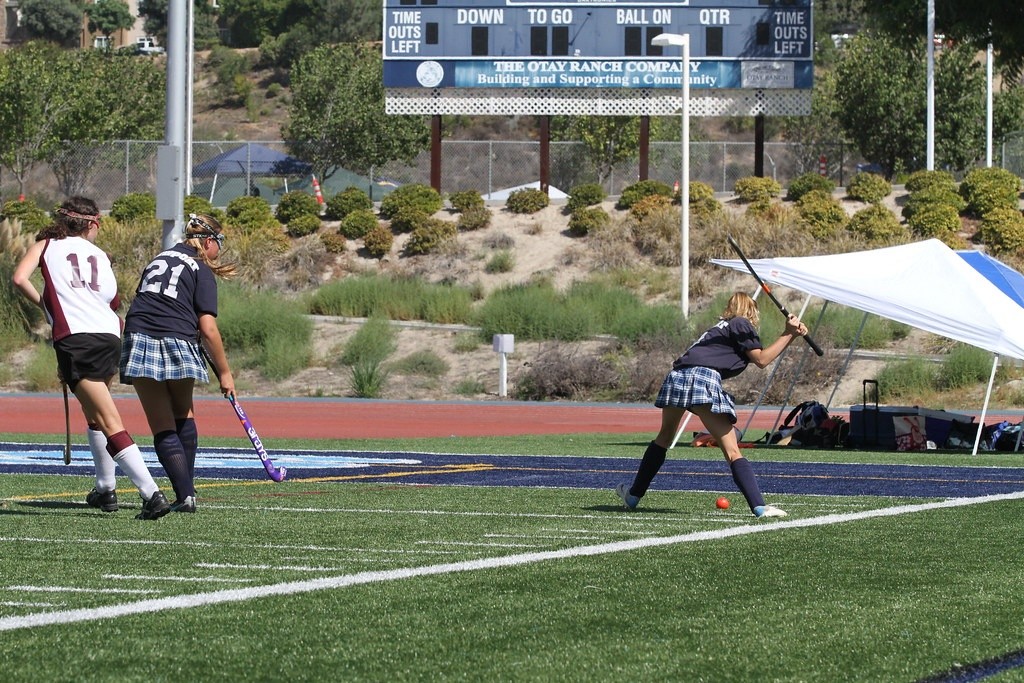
[724,233,825,356]
[715,497,730,511]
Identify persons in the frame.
[117,214,237,514]
[609,292,809,520]
[12,196,170,520]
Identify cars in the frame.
[134,41,154,52]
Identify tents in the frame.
[698,234,1024,455]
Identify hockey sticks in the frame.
[199,344,286,483]
[63,380,73,466]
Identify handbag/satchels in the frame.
[755,378,1024,454]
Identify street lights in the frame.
[649,29,695,327]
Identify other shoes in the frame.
[86,489,119,510]
[616,482,641,510]
[754,505,788,518]
[172,493,199,513]
[134,490,172,519]
